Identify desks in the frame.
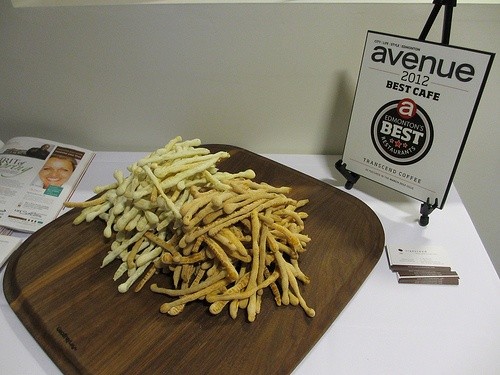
[1,149,500,375]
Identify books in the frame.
[0,134,97,232]
